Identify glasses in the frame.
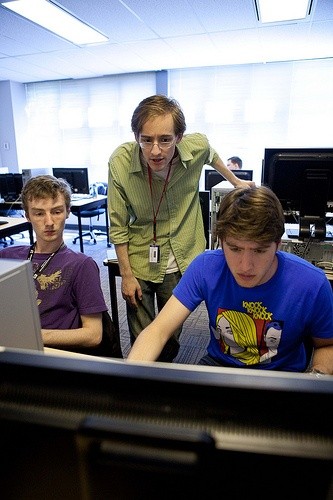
[138,134,178,150]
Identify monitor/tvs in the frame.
[52,168,89,201]
[199,191,211,250]
[204,169,253,197]
[0,173,25,202]
[0,258,43,351]
[263,148,333,239]
[0,346,333,500]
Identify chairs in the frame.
[73,182,108,245]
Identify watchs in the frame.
[305,368,326,375]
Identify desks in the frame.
[0,193,112,253]
[104,258,123,359]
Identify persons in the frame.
[127,184,333,375]
[227,156,243,170]
[0,174,109,352]
[107,93,256,363]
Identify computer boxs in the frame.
[276,222,333,270]
[209,179,237,251]
